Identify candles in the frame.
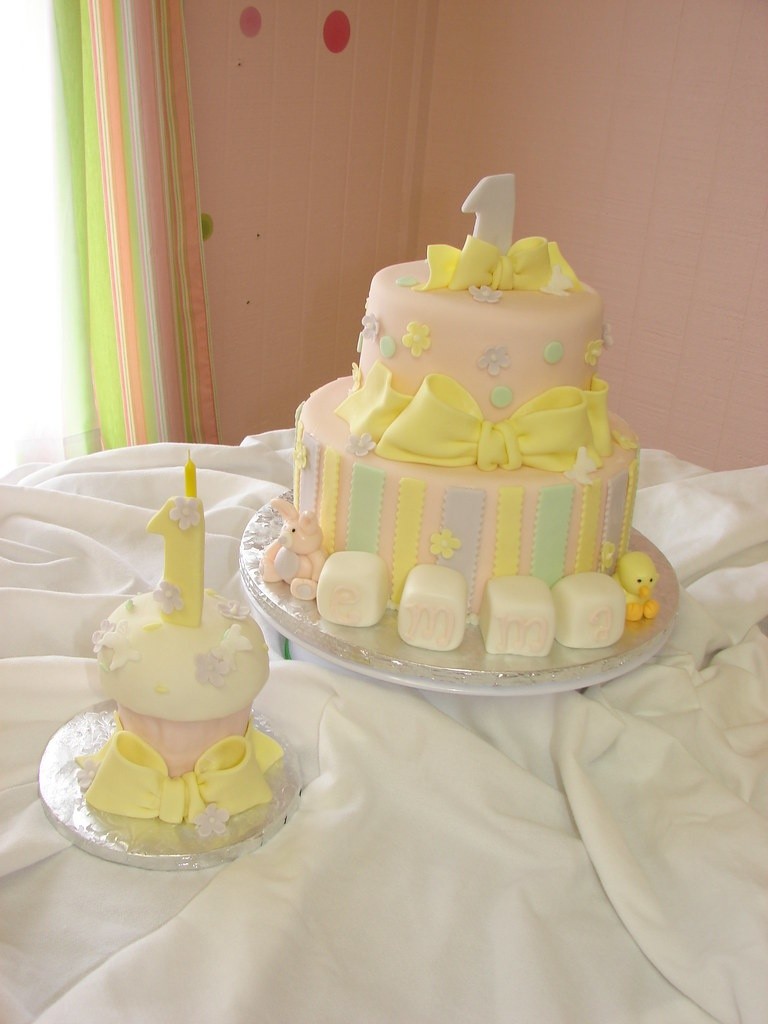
[182,445,200,498]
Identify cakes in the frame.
[74,449,285,837]
[261,173,661,659]
